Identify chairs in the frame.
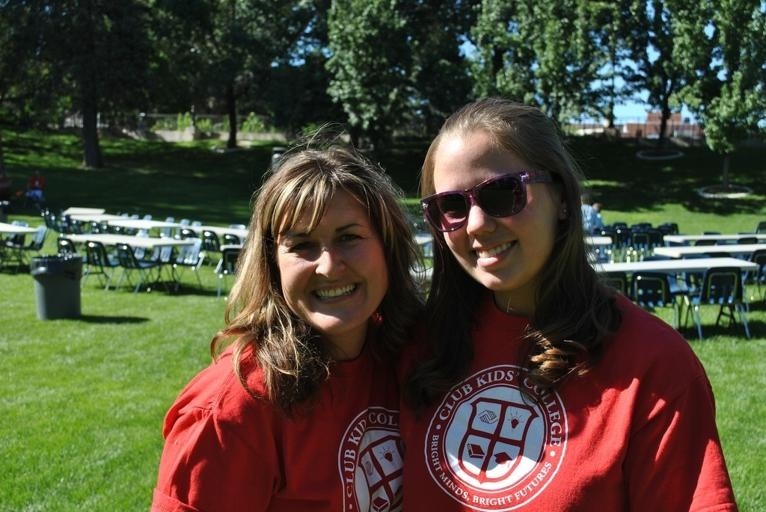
[585,220,766,342]
[0,205,250,298]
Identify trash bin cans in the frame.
[30,255,83,321]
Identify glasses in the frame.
[422,169,552,232]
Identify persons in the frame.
[150,147,425,512]
[0,169,46,206]
[392,98,738,512]
